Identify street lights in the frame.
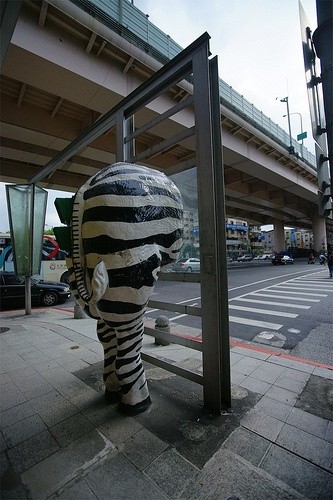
[283,113,303,145]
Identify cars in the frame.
[172,258,200,273]
[272,255,294,265]
[0,271,71,306]
[227,257,233,262]
[237,255,265,262]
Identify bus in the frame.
[0,234,77,290]
